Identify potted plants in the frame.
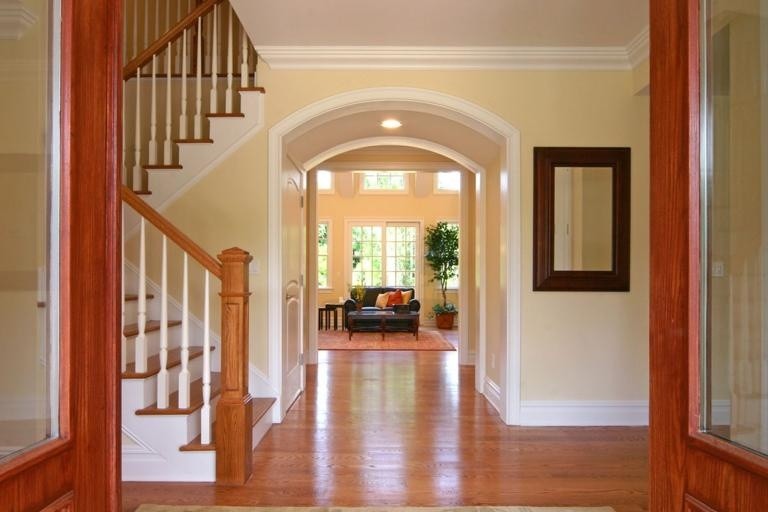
[420,221,462,331]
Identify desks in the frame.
[317,307,339,331]
[325,302,347,332]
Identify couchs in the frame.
[343,286,420,332]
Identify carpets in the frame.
[317,326,457,352]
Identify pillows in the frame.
[385,287,403,305]
[374,292,391,308]
[400,289,412,304]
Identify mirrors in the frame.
[530,144,632,292]
